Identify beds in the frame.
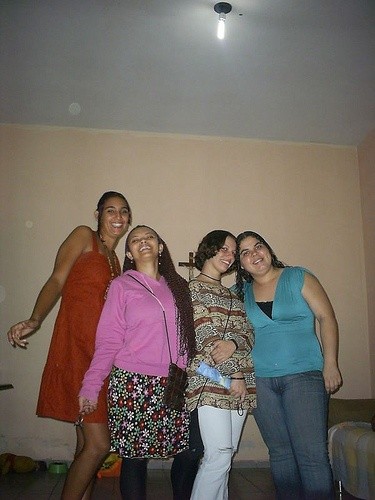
[326,397,375,500]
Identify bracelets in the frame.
[230,378,245,380]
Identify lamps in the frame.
[215,3,232,39]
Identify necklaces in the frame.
[200,273,221,282]
[97,232,121,300]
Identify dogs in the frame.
[0,453,48,475]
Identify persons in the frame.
[77,225,204,500]
[7,192,132,500]
[187,230,257,500]
[228,231,343,500]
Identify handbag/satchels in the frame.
[162,362,189,412]
